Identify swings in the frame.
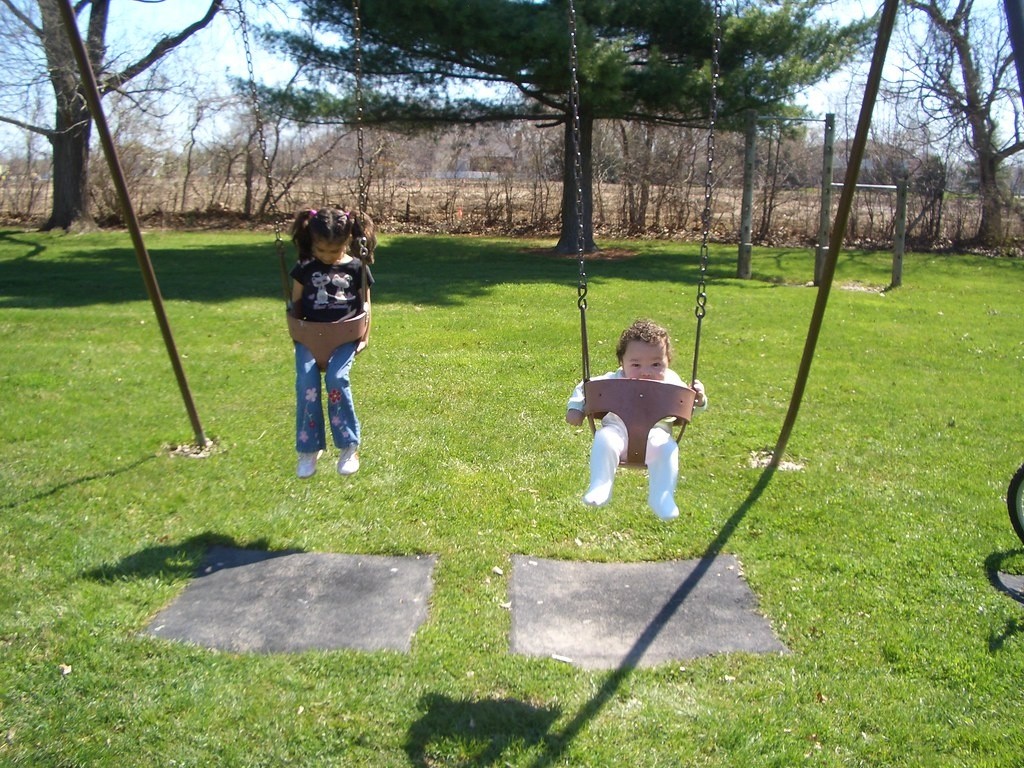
[565,0,724,472]
[233,1,372,372]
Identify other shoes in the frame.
[297,450,323,478]
[337,451,359,474]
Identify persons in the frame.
[565,317,709,518]
[288,207,377,479]
